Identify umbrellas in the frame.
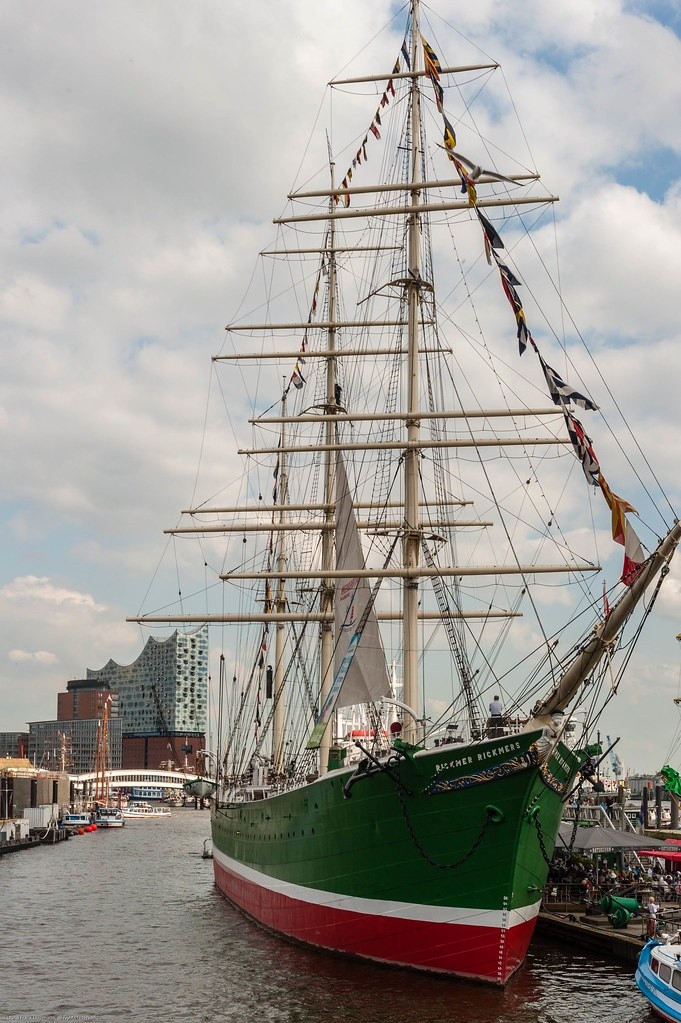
[555,825,681,888]
[555,824,584,840]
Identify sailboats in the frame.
[118,0,681,990]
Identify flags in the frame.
[292,232,330,390]
[419,35,647,590]
[333,41,410,207]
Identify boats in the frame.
[169,794,196,808]
[632,928,681,1023]
[120,800,172,818]
[94,808,126,829]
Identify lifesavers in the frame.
[635,951,641,961]
[117,814,122,819]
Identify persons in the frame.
[489,697,503,737]
[545,857,681,902]
[568,795,613,819]
[646,897,659,936]
